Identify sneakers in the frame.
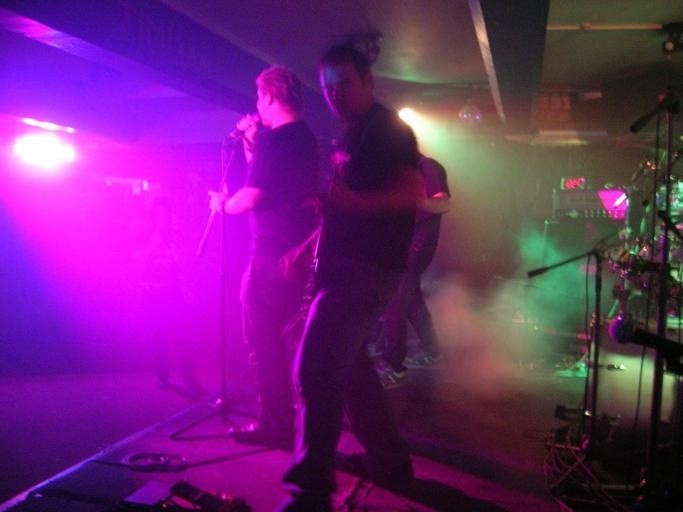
[284,489,332,512]
[378,369,410,390]
[401,349,449,371]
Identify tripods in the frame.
[171,210,257,440]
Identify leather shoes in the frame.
[230,430,296,450]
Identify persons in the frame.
[208,65,319,448]
[374,149,451,389]
[278,42,414,489]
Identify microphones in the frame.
[608,317,683,362]
[229,112,258,136]
